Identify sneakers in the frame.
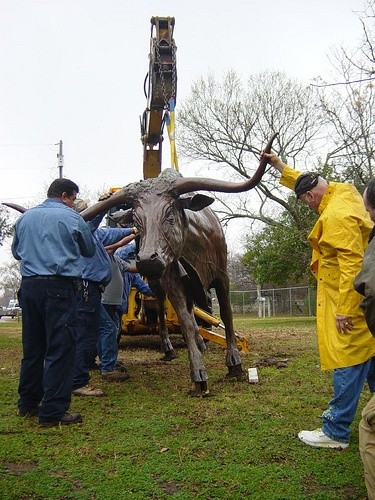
[17,360,130,425]
[298,428,349,448]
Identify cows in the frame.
[0,133,293,397]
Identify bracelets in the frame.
[133,226,139,235]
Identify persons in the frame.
[12,178,95,428]
[258,148,374,449]
[113,236,155,320]
[92,234,139,372]
[351,174,375,500]
[65,191,139,399]
[97,302,130,382]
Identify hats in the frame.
[294,172,321,199]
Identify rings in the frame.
[345,322,348,326]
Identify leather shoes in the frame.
[23,276,73,280]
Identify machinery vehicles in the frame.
[107,13,247,351]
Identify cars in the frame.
[0,297,23,319]
